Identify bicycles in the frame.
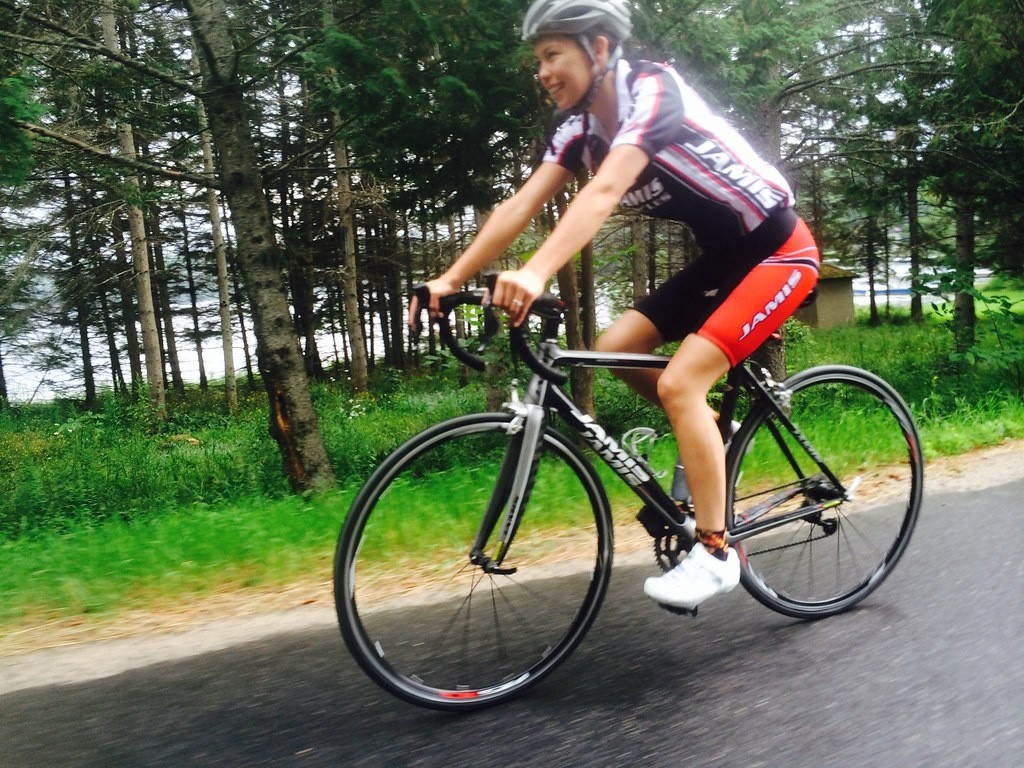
[332,269,925,716]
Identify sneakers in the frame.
[722,419,756,460]
[643,541,740,611]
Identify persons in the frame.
[405,0,821,610]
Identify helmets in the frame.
[520,0,633,44]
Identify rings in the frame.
[513,299,523,306]
